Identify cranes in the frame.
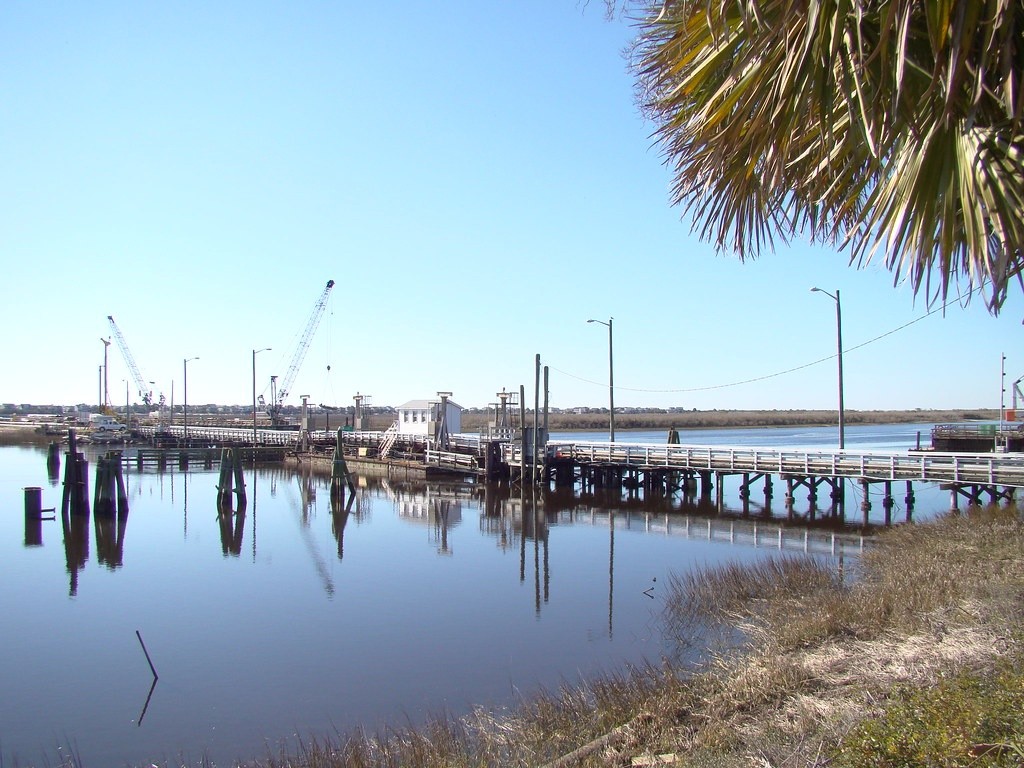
[259,279,336,427]
[101,315,167,420]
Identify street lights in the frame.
[585,318,616,456]
[182,357,200,440]
[252,348,271,447]
[810,287,848,463]
[99,365,106,413]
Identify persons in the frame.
[668,425,681,459]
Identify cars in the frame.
[90,419,127,432]
